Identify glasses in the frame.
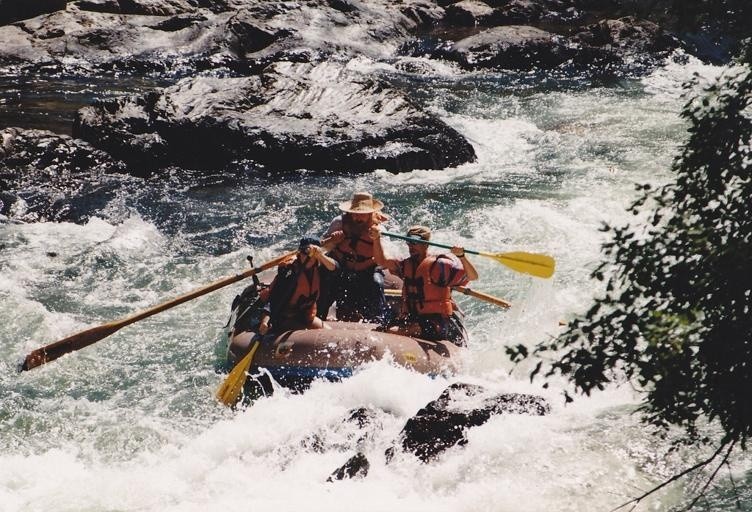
[405,238,422,245]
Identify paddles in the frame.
[216,252,310,405]
[24,234,332,371]
[381,232,555,278]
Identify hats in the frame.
[298,237,322,250]
[338,192,385,214]
[407,225,431,241]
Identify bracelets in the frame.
[456,253,465,259]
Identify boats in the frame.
[225,258,467,390]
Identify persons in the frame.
[260,234,344,329]
[318,192,391,321]
[368,227,478,338]
